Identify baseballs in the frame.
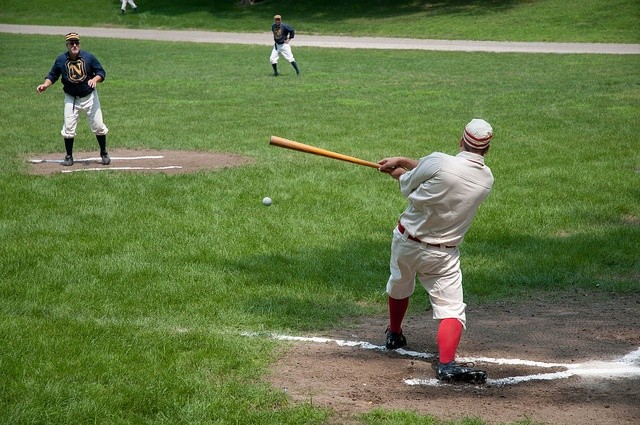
[262,197,272,206]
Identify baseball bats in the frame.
[269,135,395,172]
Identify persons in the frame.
[269,14,300,76]
[377,118,495,386]
[121,0,138,16]
[36,32,111,167]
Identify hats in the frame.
[464,117,494,149]
[274,14,281,18]
[65,31,80,42]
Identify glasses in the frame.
[68,42,80,47]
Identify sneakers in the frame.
[62,154,73,165]
[386,327,407,348]
[436,360,487,385]
[100,150,111,164]
